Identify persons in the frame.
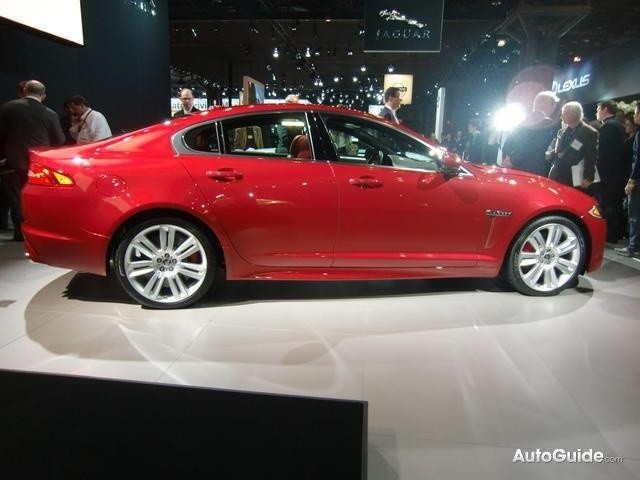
[0,79,113,242]
[432,91,640,258]
[329,104,359,157]
[372,87,409,165]
[276,92,308,153]
[174,88,217,152]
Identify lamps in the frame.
[271,47,325,88]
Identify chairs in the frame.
[290,135,311,159]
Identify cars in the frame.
[19,101,609,310]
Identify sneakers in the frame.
[615,247,639,258]
